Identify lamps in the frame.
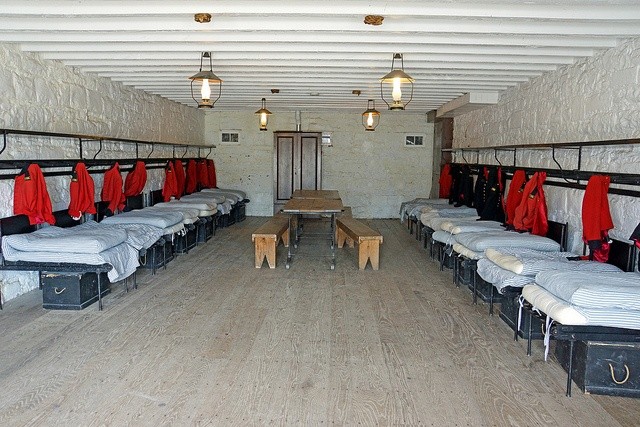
[379,52,416,111]
[254,98,272,132]
[362,100,380,132]
[189,52,224,108]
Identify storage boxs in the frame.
[421,226,435,240]
[437,244,459,270]
[231,205,253,223]
[197,216,215,242]
[41,272,115,310]
[162,224,198,253]
[139,234,178,269]
[216,206,236,229]
[456,257,477,285]
[498,290,557,340]
[430,237,441,256]
[468,267,504,303]
[555,341,640,400]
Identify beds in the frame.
[409,202,468,241]
[472,236,636,315]
[399,197,450,224]
[197,183,250,223]
[52,208,164,290]
[1,214,147,312]
[94,201,188,270]
[118,193,199,254]
[523,251,640,397]
[149,189,218,245]
[440,220,569,289]
[419,208,480,248]
[181,182,233,229]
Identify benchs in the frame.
[334,206,354,220]
[336,218,384,271]
[274,206,298,233]
[251,217,290,269]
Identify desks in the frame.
[292,189,340,235]
[282,197,343,270]
